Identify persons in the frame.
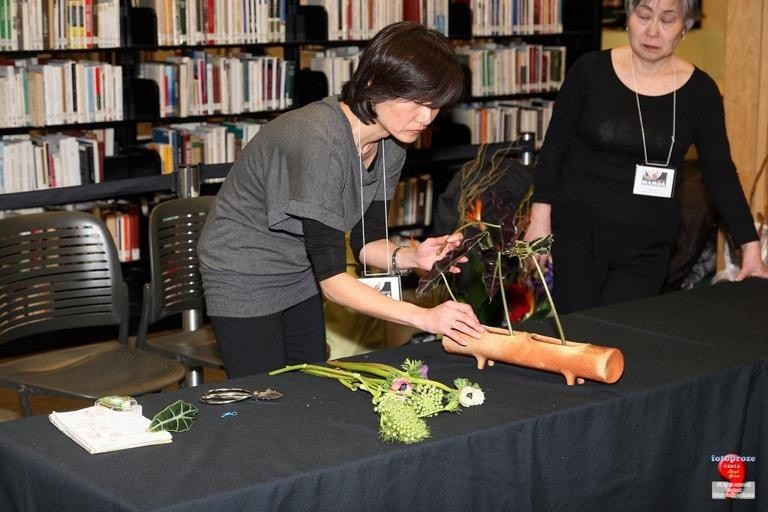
[521,1,768,317]
[196,22,488,380]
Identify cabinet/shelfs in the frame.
[1,1,607,360]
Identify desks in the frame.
[0,276,767,511]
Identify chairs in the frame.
[1,195,224,415]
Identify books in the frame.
[300,1,449,41]
[386,174,432,249]
[412,130,432,150]
[0,55,124,196]
[300,44,367,100]
[133,0,285,46]
[139,50,294,118]
[137,115,277,182]
[449,96,557,152]
[470,1,563,37]
[454,39,566,96]
[0,188,180,334]
[1,1,120,52]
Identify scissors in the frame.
[197,387,283,406]
